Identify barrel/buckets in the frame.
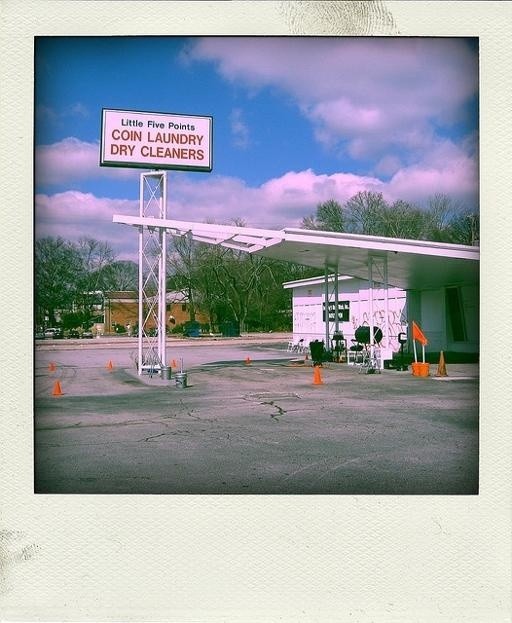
[411,362,429,377]
[161,366,171,380]
[176,373,187,389]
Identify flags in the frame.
[412,320,427,344]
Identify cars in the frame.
[82,329,93,338]
[68,330,80,338]
[53,331,64,338]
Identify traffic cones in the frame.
[49,362,57,372]
[52,380,65,396]
[171,359,176,367]
[108,359,113,369]
[246,356,250,365]
[311,366,323,385]
[305,353,309,360]
[434,349,448,377]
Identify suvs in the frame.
[42,327,61,337]
